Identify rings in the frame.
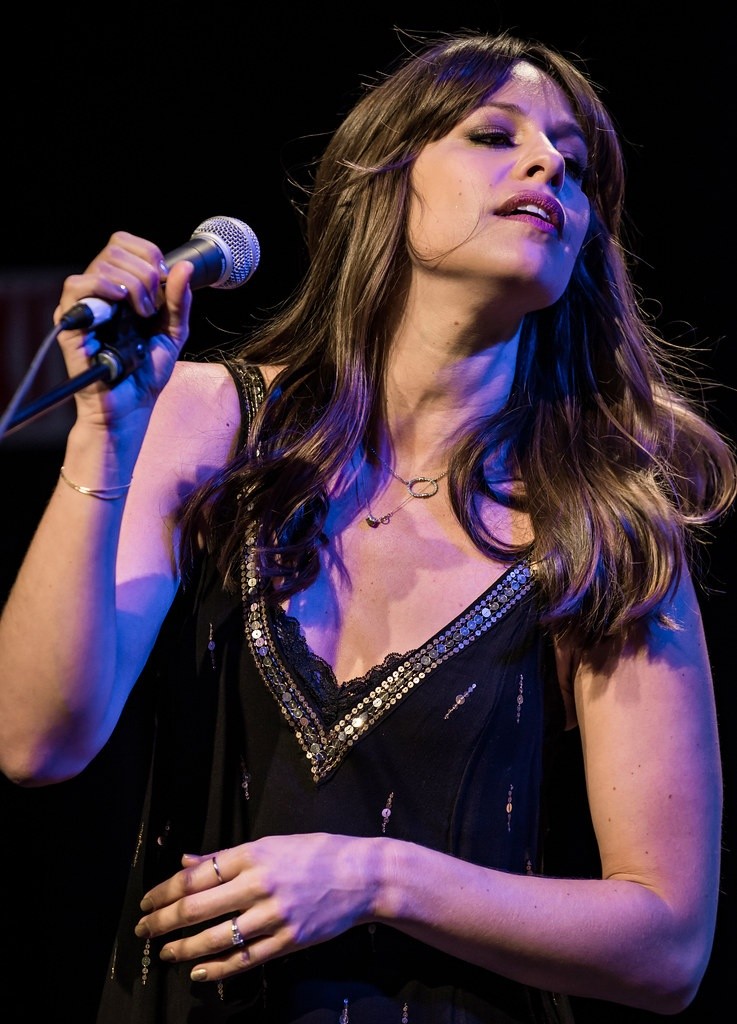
[213,856,224,882]
[232,917,244,947]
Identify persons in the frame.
[0,35,737,1023]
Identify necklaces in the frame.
[357,446,447,528]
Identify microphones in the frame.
[60,216,260,330]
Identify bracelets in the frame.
[60,467,134,500]
